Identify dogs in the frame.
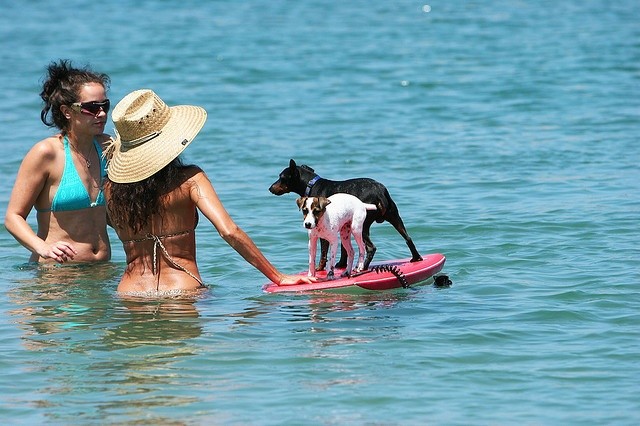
[269,158,423,270]
[296,192,376,281]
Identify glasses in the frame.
[65,99,110,116]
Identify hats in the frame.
[99,88,208,185]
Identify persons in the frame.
[3,57,114,266]
[102,86,318,296]
